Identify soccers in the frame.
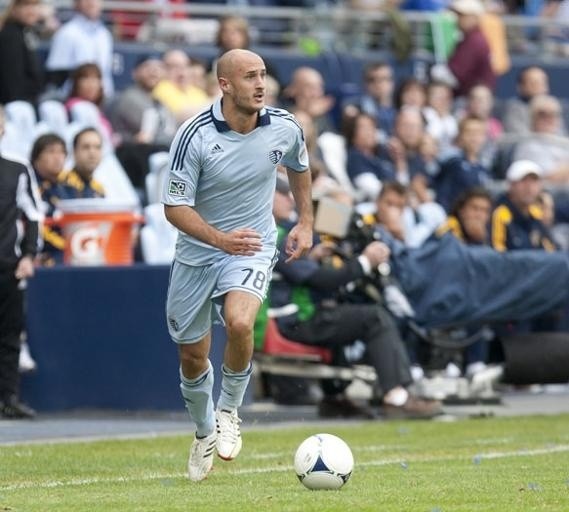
[293,432,354,490]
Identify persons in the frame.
[159,47,314,481]
[1,0,569,423]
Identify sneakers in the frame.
[188,429,217,480]
[216,407,243,460]
[1,397,37,418]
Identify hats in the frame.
[506,160,542,181]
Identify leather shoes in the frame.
[381,395,443,420]
